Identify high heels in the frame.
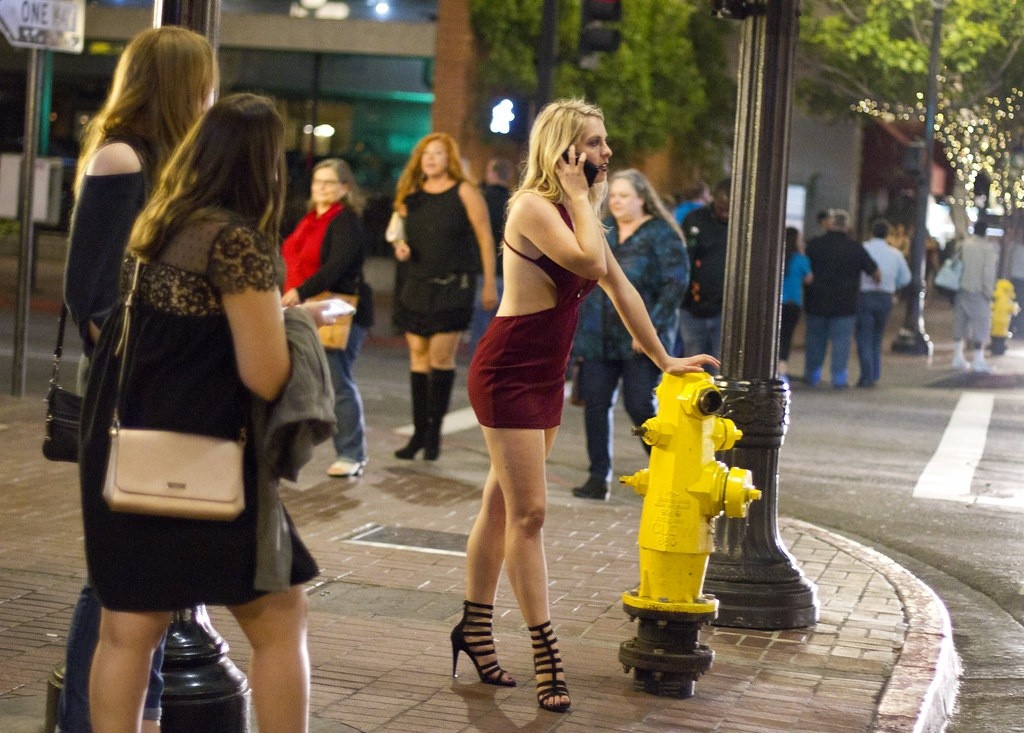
[450,599,516,686]
[528,620,571,709]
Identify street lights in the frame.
[894,1,955,356]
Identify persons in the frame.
[387,132,500,465]
[658,169,733,374]
[562,161,692,502]
[949,218,1000,375]
[281,157,375,478]
[885,223,942,288]
[458,154,516,358]
[88,92,338,733]
[777,225,814,376]
[58,27,224,733]
[803,205,882,389]
[452,96,721,713]
[851,216,912,389]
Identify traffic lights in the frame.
[579,1,625,73]
[477,89,533,144]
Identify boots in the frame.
[395,368,457,460]
[409,371,441,461]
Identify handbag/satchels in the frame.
[43,388,83,463]
[101,427,247,521]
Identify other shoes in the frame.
[326,455,370,478]
[572,477,611,500]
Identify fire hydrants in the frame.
[617,362,762,694]
[979,278,1024,359]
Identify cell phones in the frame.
[562,149,598,188]
[284,299,356,318]
